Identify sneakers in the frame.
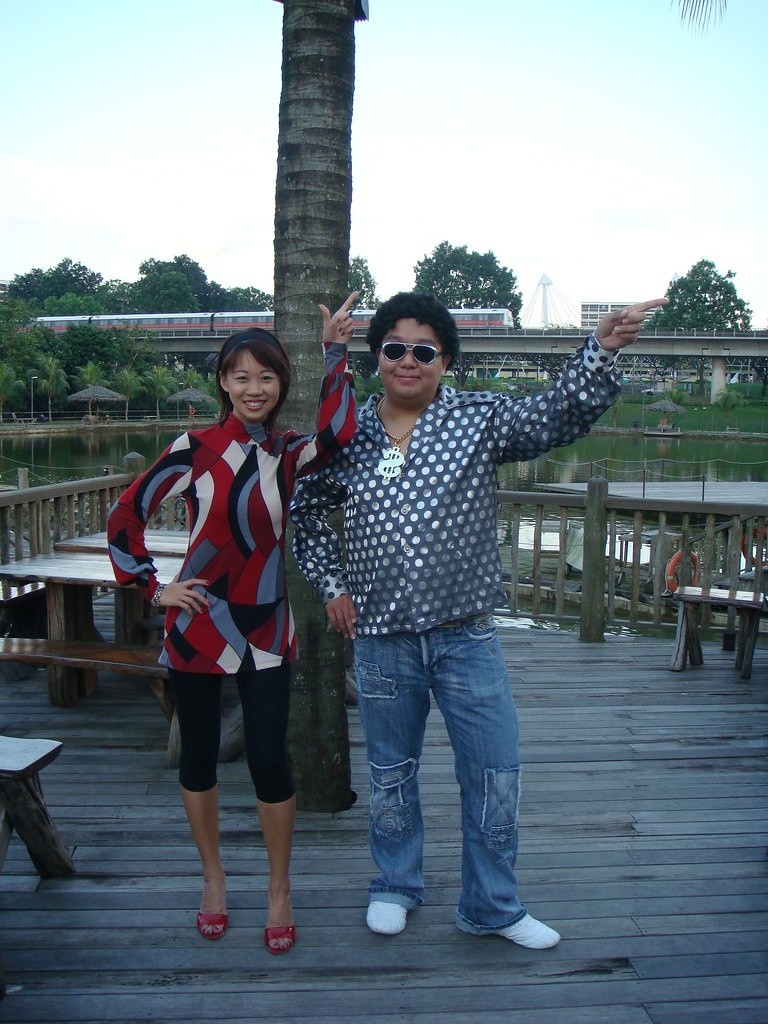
[497,914,560,949]
[366,901,408,934]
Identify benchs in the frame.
[1,637,175,721]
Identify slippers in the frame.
[264,924,296,954]
[197,911,229,939]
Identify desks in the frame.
[0,530,364,770]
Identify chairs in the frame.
[669,509,768,679]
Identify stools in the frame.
[0,736,78,881]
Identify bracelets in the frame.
[152,584,166,608]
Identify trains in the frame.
[3,308,513,338]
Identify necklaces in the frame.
[374,389,432,483]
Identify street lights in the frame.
[30,377,38,420]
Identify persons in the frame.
[103,288,374,956]
[660,415,667,433]
[293,288,678,950]
[189,404,196,422]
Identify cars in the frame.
[642,387,666,395]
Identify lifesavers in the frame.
[664,550,701,592]
[740,527,768,565]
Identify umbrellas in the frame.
[167,386,215,407]
[68,385,129,416]
[642,400,687,418]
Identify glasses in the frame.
[380,341,443,365]
